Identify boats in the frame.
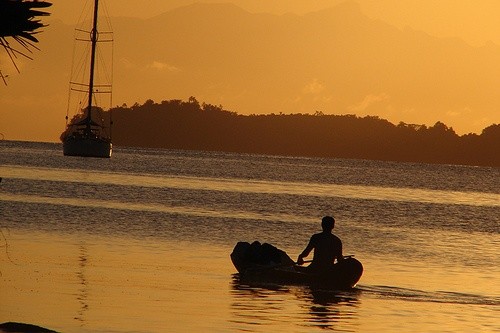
[230,237,364,292]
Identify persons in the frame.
[296,215,345,270]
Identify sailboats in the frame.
[58,0,115,158]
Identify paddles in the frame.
[294,254,356,264]
